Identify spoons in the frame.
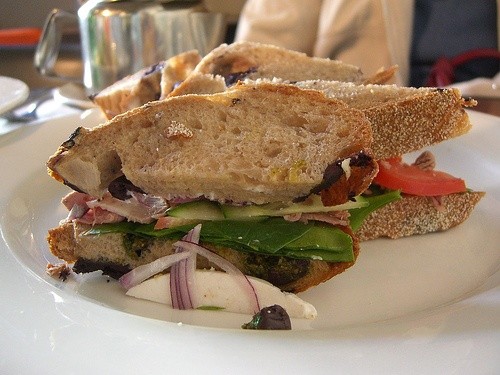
[7,91,51,124]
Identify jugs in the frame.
[33,0,228,97]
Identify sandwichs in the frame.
[40,41,487,334]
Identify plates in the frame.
[51,83,96,109]
[1,72,29,115]
[0,105,500,338]
